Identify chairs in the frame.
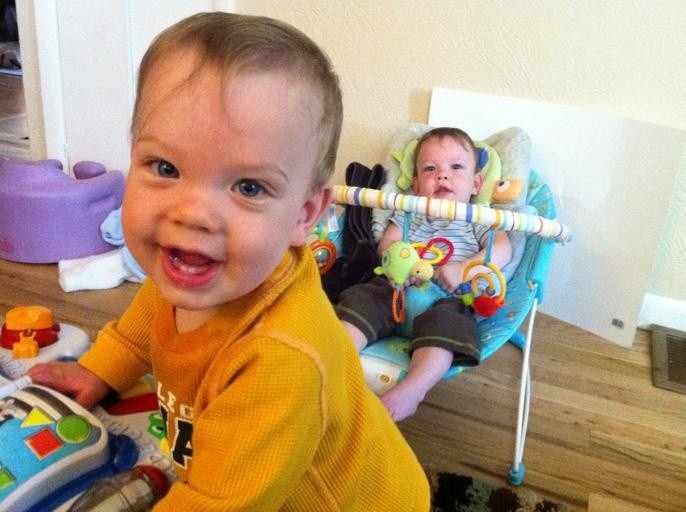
[329,166,573,485]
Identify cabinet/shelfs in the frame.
[0,0,47,163]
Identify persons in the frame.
[337,128,512,423]
[29,13,432,512]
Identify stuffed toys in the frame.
[374,236,456,324]
